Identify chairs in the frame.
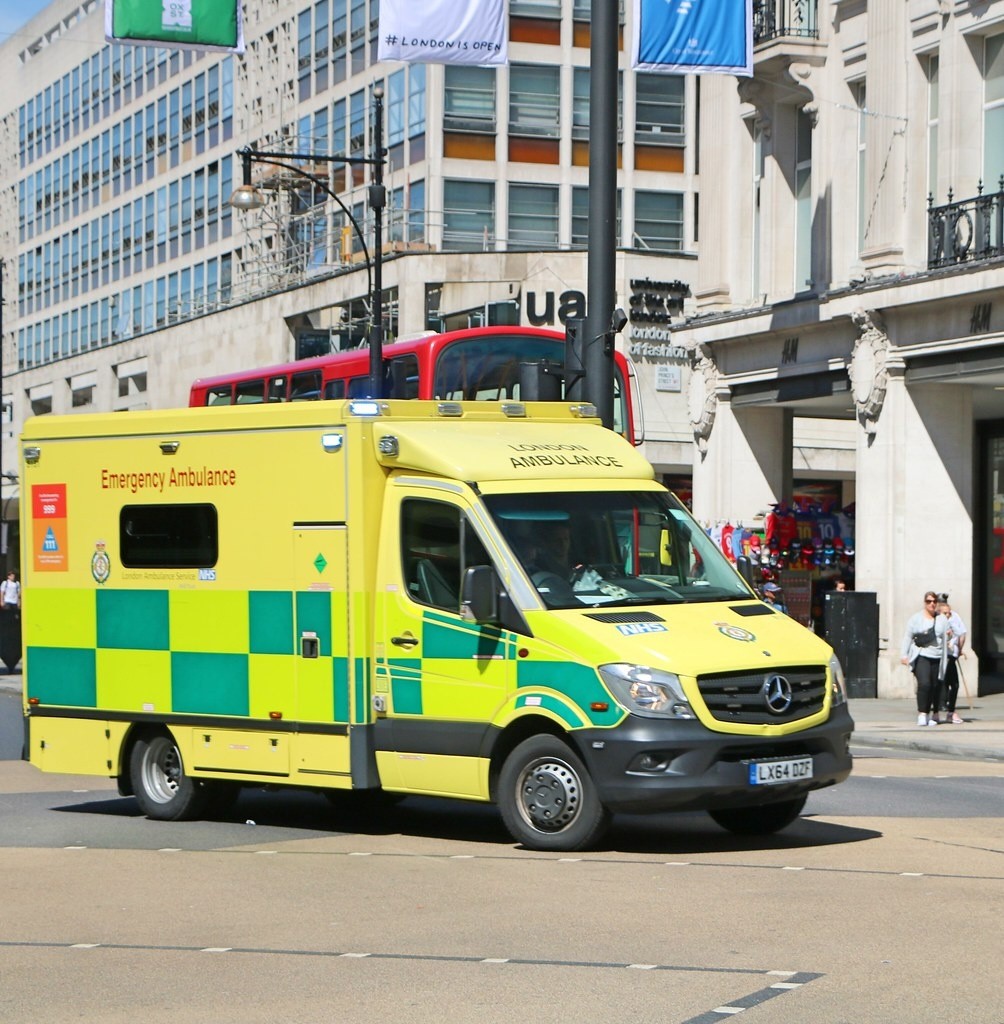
[413,516,460,613]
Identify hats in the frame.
[763,582,782,593]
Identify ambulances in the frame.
[19,399,854,854]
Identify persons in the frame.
[901,591,954,726]
[835,582,846,592]
[527,525,599,592]
[1,572,21,608]
[764,583,787,615]
[934,593,967,723]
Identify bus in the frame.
[190,324,642,577]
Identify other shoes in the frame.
[930,705,949,712]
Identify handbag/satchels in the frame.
[912,626,937,648]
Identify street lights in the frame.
[228,150,384,398]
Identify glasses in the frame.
[942,611,951,616]
[925,600,938,605]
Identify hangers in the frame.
[698,499,855,531]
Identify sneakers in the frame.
[917,712,937,726]
[947,713,963,724]
[932,711,939,724]
